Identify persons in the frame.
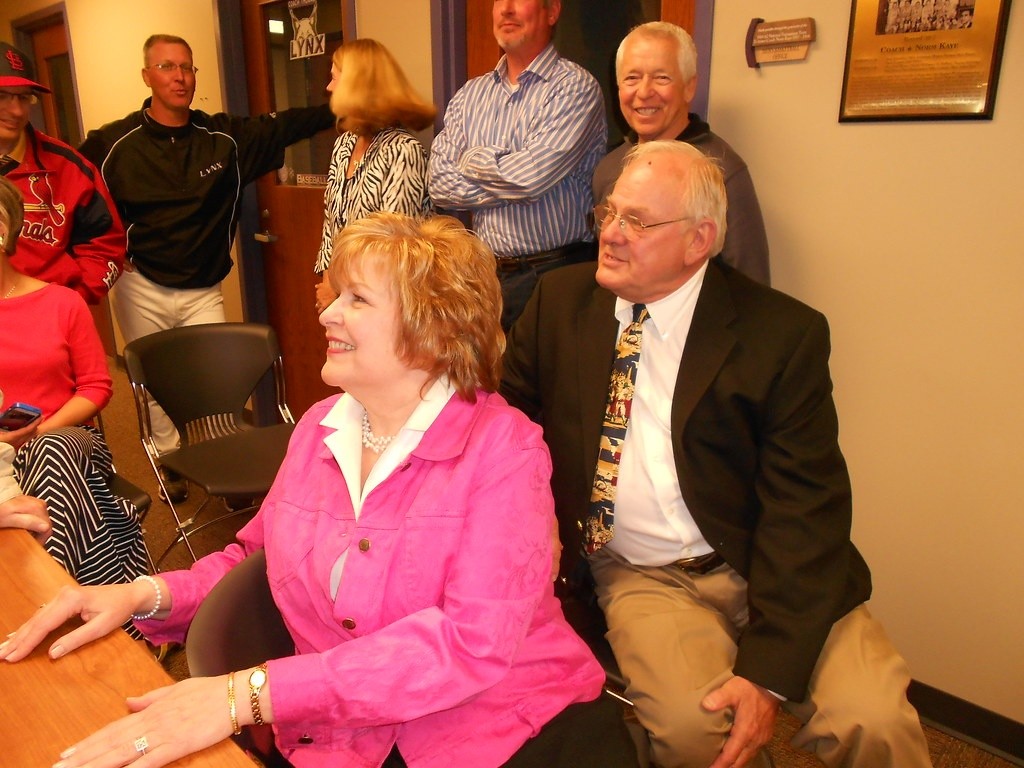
[0,41,128,306]
[425,0,607,335]
[590,21,771,288]
[497,141,933,768]
[310,38,440,316]
[0,180,176,663]
[78,35,338,513]
[0,214,643,768]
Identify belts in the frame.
[670,551,724,575]
[495,246,568,277]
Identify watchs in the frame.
[249,662,269,726]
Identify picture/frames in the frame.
[841,0,1013,123]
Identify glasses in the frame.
[146,62,199,74]
[0,90,40,104]
[590,206,692,240]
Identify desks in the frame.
[1,527,267,768]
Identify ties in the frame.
[582,304,653,555]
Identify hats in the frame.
[0,42,52,94]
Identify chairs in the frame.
[124,323,300,570]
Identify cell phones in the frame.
[0,403,40,431]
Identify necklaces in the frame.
[361,408,394,453]
[353,147,363,175]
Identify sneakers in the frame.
[157,464,189,504]
[223,493,252,511]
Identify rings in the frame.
[39,601,47,609]
[135,736,148,756]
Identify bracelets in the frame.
[129,575,161,620]
[228,672,242,735]
